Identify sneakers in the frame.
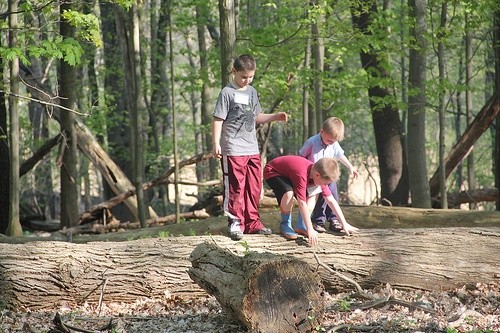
[329,219,344,233]
[313,219,327,233]
[227,217,243,240]
[254,227,272,234]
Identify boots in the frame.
[279,211,298,239]
[295,209,308,237]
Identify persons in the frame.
[212,55,288,241]
[263,155,359,247]
[299,117,359,233]
